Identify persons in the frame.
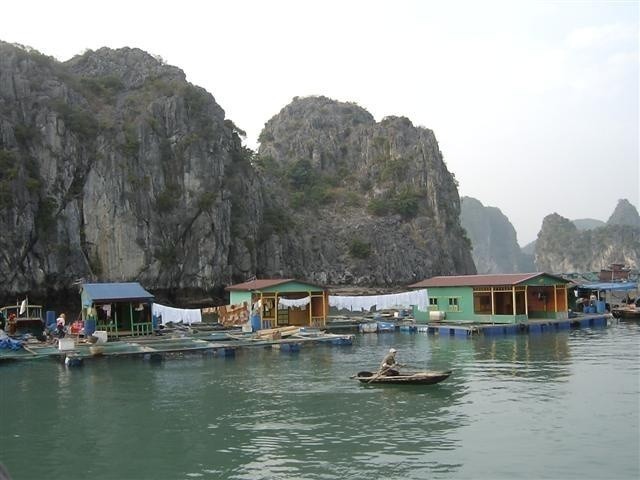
[56,313,65,338]
[381,348,404,376]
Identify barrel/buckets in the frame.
[47,310,56,325]
[597,301,604,314]
[251,315,261,330]
[84,320,95,335]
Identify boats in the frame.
[355,368,451,385]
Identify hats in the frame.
[390,348,397,353]
